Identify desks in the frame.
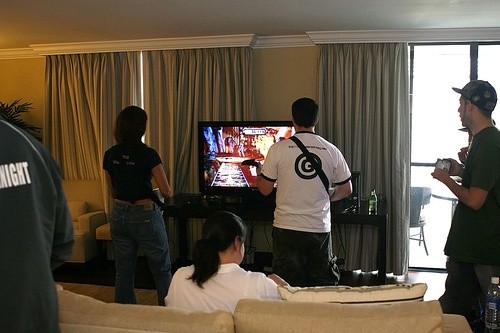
[164,193,388,286]
[431,194,459,218]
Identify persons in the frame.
[431,80,500,333]
[238,133,245,157]
[164,211,289,313]
[103,105,172,306]
[256,97,352,286]
[0,118,75,333]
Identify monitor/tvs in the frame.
[197,120,296,198]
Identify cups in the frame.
[435,159,451,173]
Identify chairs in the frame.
[409,187,431,256]
[62,179,112,284]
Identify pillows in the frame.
[67,200,87,221]
[277,283,427,305]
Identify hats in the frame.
[452,80,497,112]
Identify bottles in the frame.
[485,277,500,330]
[368,189,378,216]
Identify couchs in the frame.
[53,284,473,333]
